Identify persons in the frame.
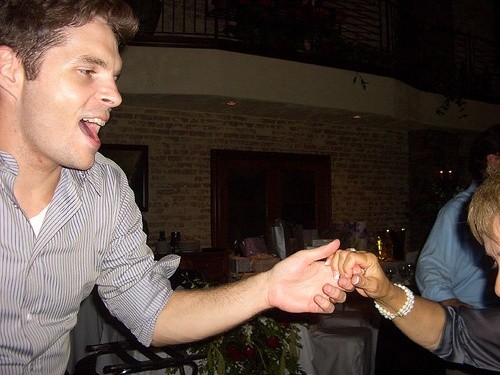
[0,1,364,375]
[323,168,499,374]
[414,122,500,309]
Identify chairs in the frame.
[300,242,433,375]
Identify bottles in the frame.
[170,231,182,255]
[157,230,168,254]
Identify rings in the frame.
[346,248,357,254]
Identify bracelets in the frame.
[374,281,415,321]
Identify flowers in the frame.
[165,269,306,375]
